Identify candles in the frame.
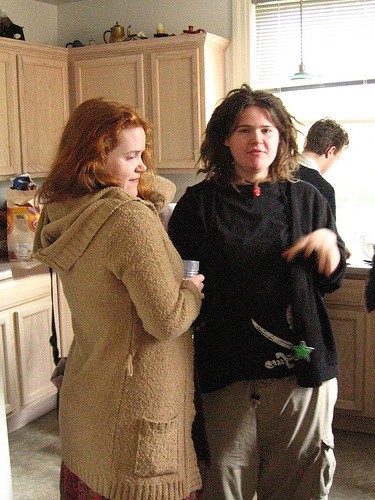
[157,22,164,33]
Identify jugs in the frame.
[182,25,205,34]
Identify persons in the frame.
[166,84,347,500]
[36,97,204,500]
[293,119,351,259]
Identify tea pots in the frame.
[103,21,131,44]
[66,40,85,49]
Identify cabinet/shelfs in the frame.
[323,279,375,433]
[0,272,74,435]
[0,31,232,183]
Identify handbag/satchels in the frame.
[6,173,43,261]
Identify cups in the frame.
[182,260,200,283]
[89,39,96,45]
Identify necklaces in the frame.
[239,173,271,197]
